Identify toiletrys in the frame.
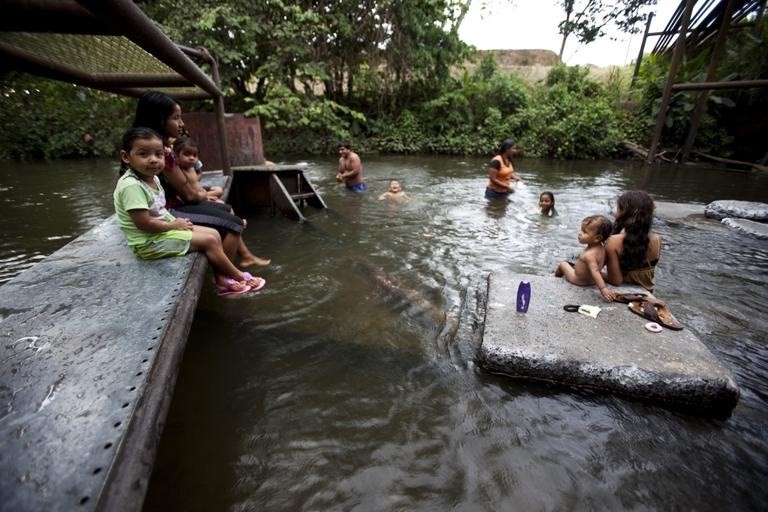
[516,281,531,313]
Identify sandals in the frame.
[213,271,268,298]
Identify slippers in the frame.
[630,300,685,332]
[607,289,649,304]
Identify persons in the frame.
[335,142,366,192]
[113,92,272,301]
[484,138,517,195]
[538,192,559,217]
[555,216,615,303]
[379,180,412,203]
[604,190,660,294]
